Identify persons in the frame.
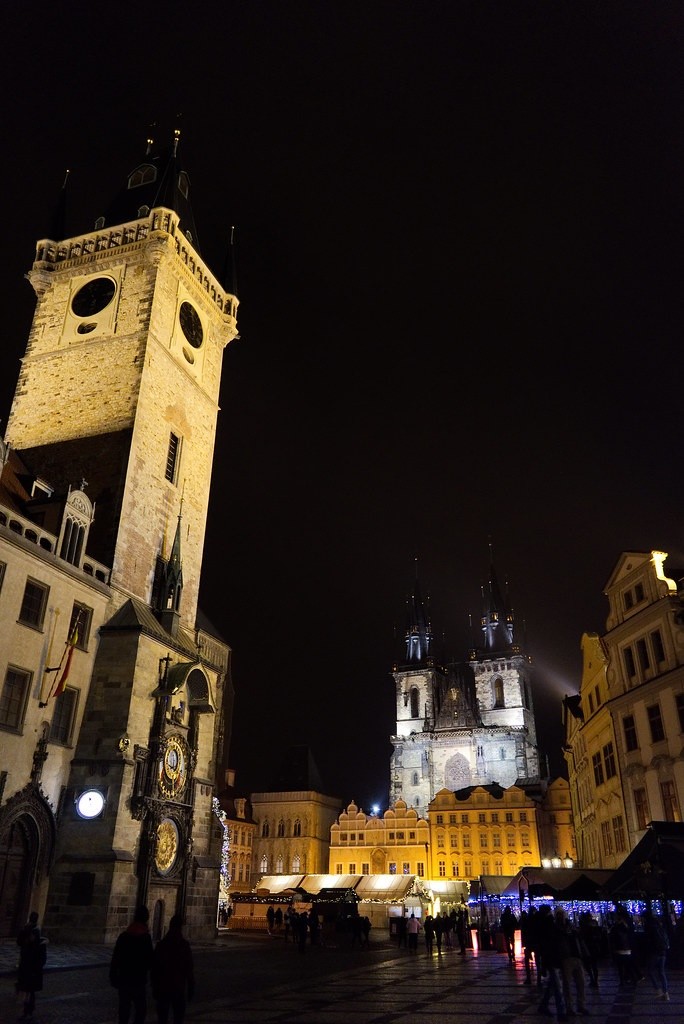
[499,899,684,1024]
[266,906,371,946]
[14,911,48,1016]
[151,915,193,1024]
[218,906,233,925]
[397,908,468,958]
[110,905,153,1024]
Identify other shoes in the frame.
[633,975,646,985]
[654,993,664,1000]
[665,992,670,1001]
[576,1008,589,1014]
[524,980,531,984]
[619,983,624,987]
[537,984,544,992]
[589,982,598,987]
[538,1006,554,1017]
[566,1008,576,1015]
[557,1014,568,1020]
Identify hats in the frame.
[135,905,149,922]
[171,913,183,925]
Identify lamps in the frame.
[541,852,551,868]
[563,851,573,867]
[551,851,561,868]
